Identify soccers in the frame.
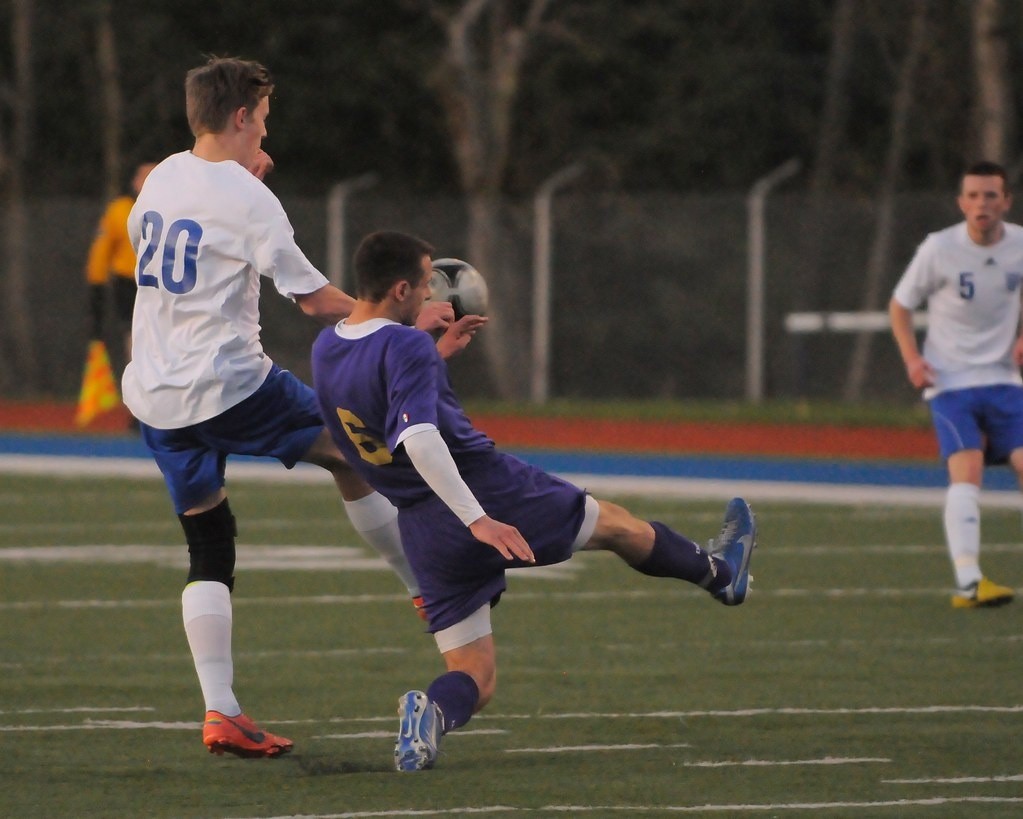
[422,256,489,321]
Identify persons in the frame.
[312,230,754,770]
[889,161,1023,610]
[87,154,160,402]
[120,58,458,757]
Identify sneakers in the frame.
[203,707,294,760]
[412,595,428,622]
[952,577,1016,609]
[705,496,759,606]
[394,690,445,772]
